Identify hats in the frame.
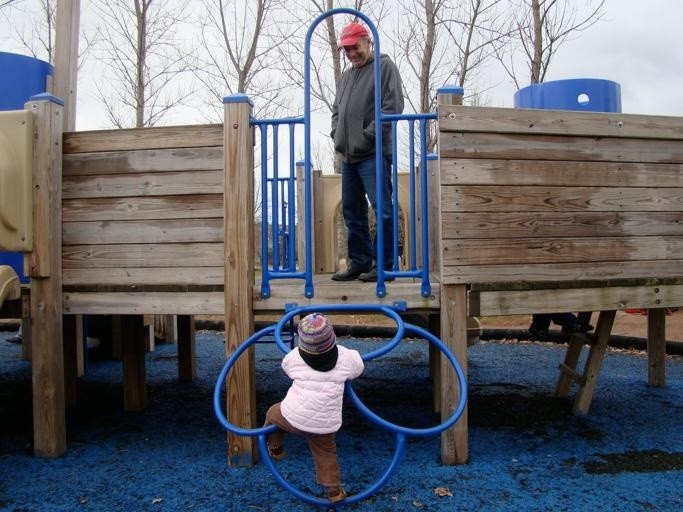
[298,313,337,355]
[337,22,368,51]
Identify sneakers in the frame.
[267,441,285,462]
[327,487,345,503]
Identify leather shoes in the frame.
[357,265,395,281]
[331,264,361,280]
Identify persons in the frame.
[366,182,405,281]
[263,313,364,503]
[6,323,22,345]
[529,313,594,334]
[329,23,404,283]
